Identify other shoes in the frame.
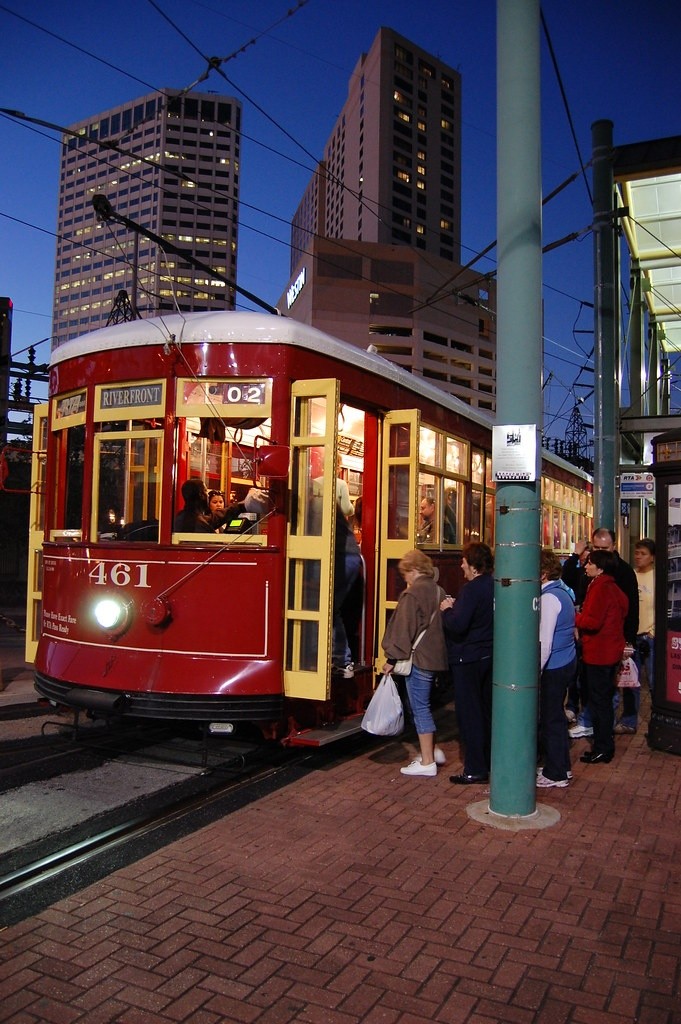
[580,751,614,763]
[564,709,577,723]
[568,725,594,738]
[535,767,573,779]
[535,775,569,787]
[449,773,488,784]
[612,722,636,735]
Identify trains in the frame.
[23,305,593,773]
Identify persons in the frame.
[535,548,576,788]
[175,478,247,533]
[560,527,640,767]
[382,551,449,776]
[312,453,363,677]
[613,537,654,737]
[419,488,459,544]
[438,540,491,786]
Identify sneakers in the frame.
[400,760,437,776]
[331,662,354,679]
[414,749,445,765]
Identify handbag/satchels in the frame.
[613,647,642,688]
[392,652,414,676]
[360,673,405,737]
